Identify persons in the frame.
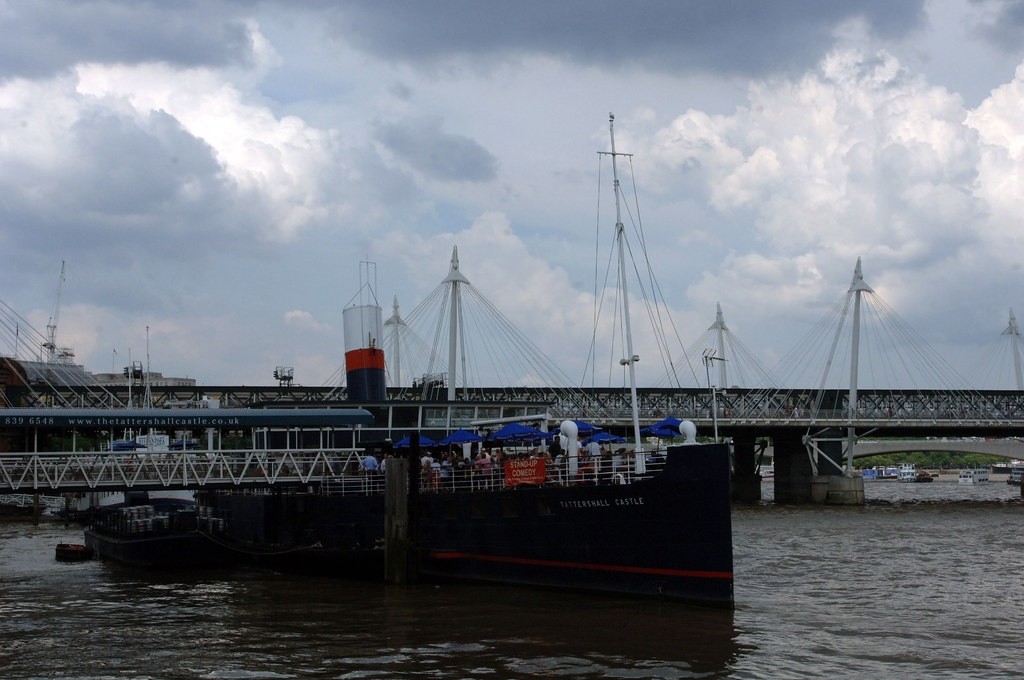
[357,435,661,484]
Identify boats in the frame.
[55,542,94,562]
[958,468,991,486]
[1008,470,1024,485]
[896,462,917,483]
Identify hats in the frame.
[427,451,433,455]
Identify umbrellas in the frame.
[112,439,198,453]
[391,415,682,454]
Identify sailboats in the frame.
[83,108,735,611]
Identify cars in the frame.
[170,439,200,450]
[108,440,147,452]
